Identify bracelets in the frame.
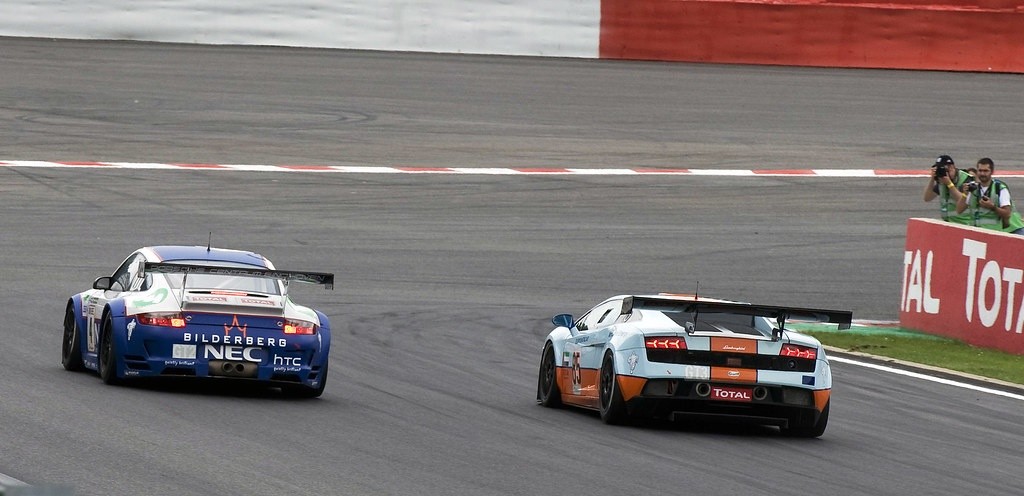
[947,183,955,188]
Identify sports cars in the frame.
[535,289,857,438]
[58,246,333,400]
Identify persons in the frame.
[922,155,976,228]
[957,157,1023,235]
[967,168,979,177]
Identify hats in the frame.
[933,156,954,167]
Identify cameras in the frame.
[963,181,980,191]
[935,165,948,181]
